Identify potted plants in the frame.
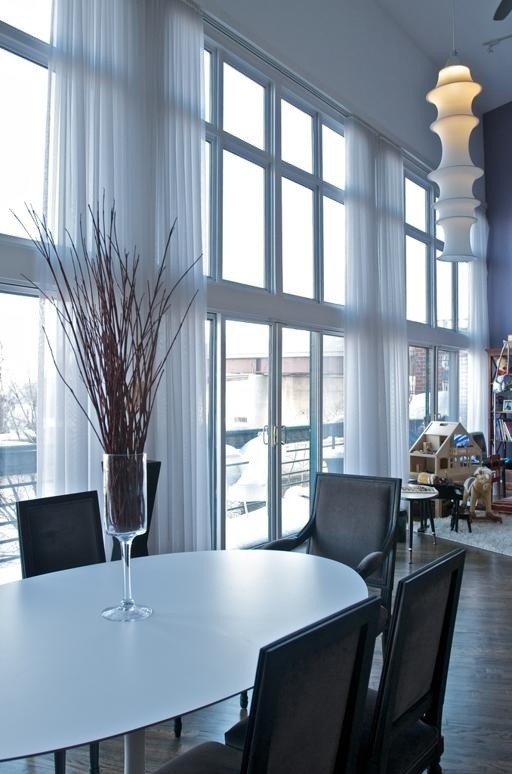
[10,199,205,621]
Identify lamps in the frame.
[425,25,486,262]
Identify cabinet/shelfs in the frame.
[485,347,512,515]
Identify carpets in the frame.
[406,499,512,557]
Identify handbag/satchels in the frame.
[492,374,512,394]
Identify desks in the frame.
[400,483,439,564]
[1,549,368,774]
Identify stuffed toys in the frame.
[457,466,502,523]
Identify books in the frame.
[495,412,512,442]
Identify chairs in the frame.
[241,472,401,709]
[15,489,181,774]
[224,548,465,774]
[154,594,387,774]
[469,432,505,502]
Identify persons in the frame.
[504,401,511,411]
